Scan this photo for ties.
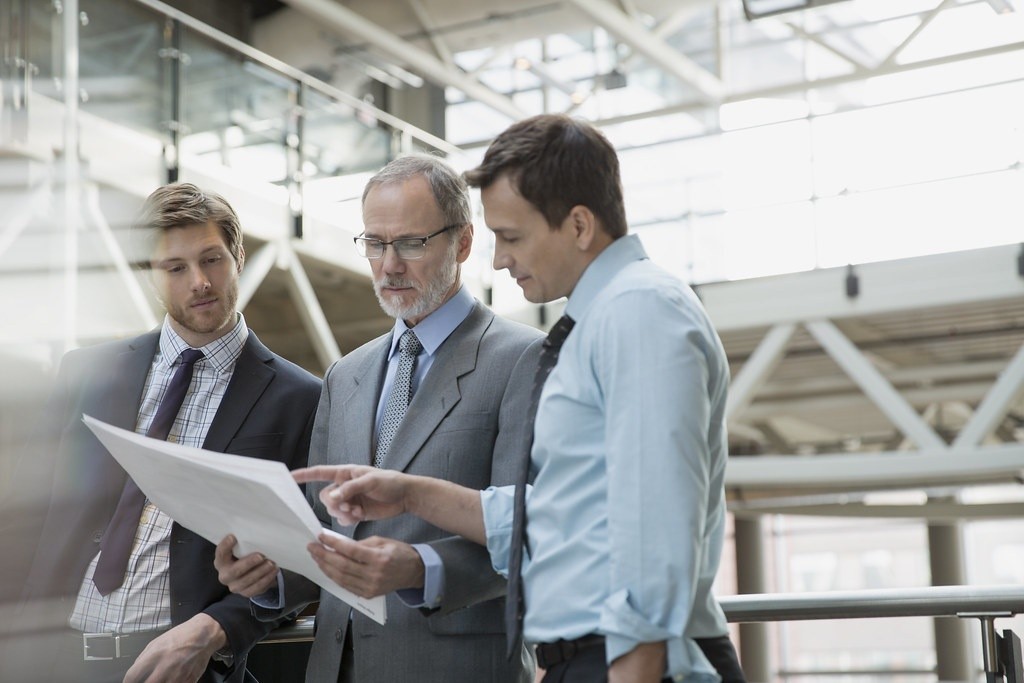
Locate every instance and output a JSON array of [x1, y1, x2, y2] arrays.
[[504, 316, 576, 662], [92, 349, 206, 598], [371, 329, 423, 468]]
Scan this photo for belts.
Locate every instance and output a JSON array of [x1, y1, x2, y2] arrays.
[[59, 628, 172, 663], [536, 637, 605, 668]]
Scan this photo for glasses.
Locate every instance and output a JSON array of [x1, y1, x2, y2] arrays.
[[355, 223, 458, 259]]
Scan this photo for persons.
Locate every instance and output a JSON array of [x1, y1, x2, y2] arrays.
[[214, 154, 550, 682], [290, 113, 741, 682], [23, 113, 323, 683]]
